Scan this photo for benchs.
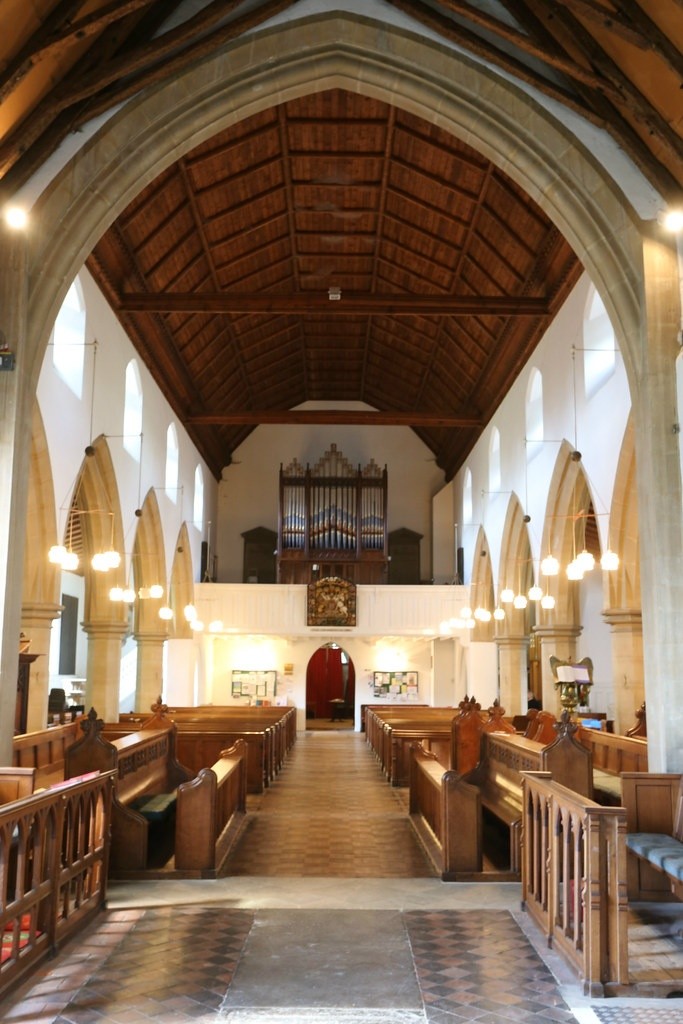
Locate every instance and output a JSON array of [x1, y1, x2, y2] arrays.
[[363, 706, 514, 786], [0, 695, 195, 883], [450, 694, 647, 872], [619, 772, 683, 902], [77, 706, 297, 793]]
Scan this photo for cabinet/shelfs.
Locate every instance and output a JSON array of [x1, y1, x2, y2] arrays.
[[240, 526, 277, 584], [389, 527, 425, 585]]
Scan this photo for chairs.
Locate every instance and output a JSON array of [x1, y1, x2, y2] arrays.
[[48, 688, 68, 725]]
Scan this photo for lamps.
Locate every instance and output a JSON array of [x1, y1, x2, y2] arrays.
[[44, 337, 205, 635], [441, 343, 622, 634]]
